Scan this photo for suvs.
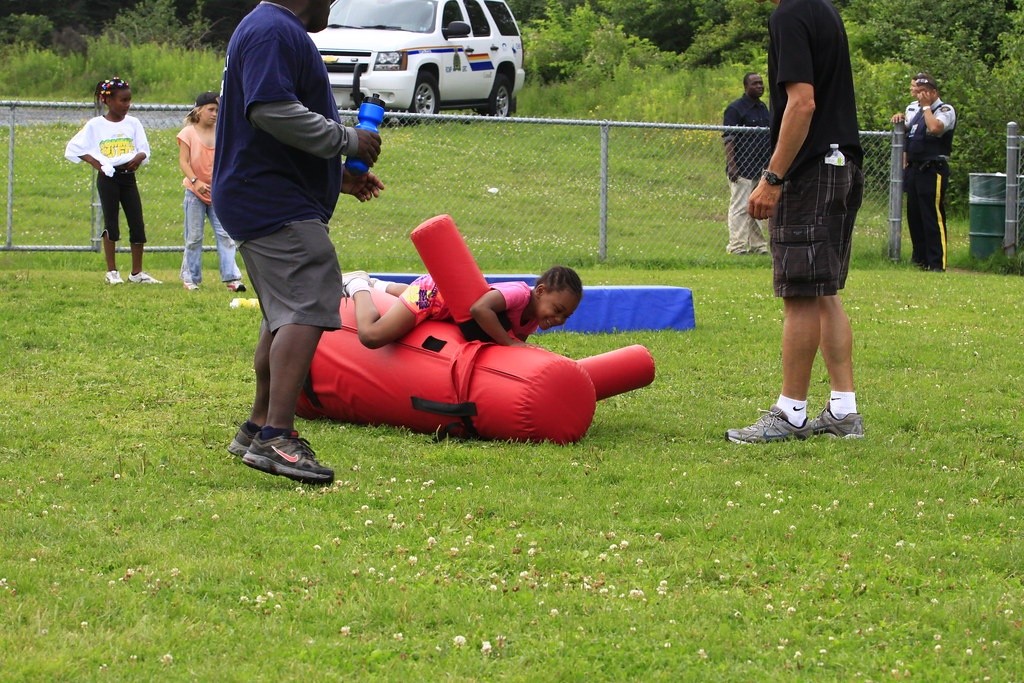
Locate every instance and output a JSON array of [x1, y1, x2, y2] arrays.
[[306, 1, 525, 125]]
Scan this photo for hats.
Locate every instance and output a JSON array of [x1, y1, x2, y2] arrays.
[[186, 91, 221, 117]]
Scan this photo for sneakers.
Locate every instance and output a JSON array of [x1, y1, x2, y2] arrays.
[[105, 270, 125, 286], [342, 271, 370, 296], [226, 280, 246, 292], [183, 281, 200, 290], [227, 422, 256, 457], [810, 400, 865, 439], [724, 403, 814, 443], [242, 428, 335, 486], [126, 272, 163, 285]]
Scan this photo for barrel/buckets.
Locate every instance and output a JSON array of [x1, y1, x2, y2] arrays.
[[969, 173, 1024, 262]]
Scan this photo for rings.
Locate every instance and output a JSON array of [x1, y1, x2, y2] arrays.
[[201, 189, 206, 195]]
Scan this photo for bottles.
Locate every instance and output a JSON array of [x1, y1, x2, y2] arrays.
[[825, 145, 845, 167], [230, 298, 260, 309], [344, 93, 385, 174]]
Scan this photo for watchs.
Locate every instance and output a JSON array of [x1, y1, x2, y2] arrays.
[[191, 177, 198, 183], [762, 170, 785, 186]]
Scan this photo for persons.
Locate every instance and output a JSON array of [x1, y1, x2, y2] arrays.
[[723, 71, 773, 255], [342, 266, 584, 350], [177, 90, 247, 292], [210, 0, 385, 483], [65, 76, 162, 284], [722, 0, 870, 446], [889, 73, 955, 271]]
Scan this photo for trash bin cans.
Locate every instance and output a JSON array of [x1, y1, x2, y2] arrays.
[[968, 168, 1021, 272]]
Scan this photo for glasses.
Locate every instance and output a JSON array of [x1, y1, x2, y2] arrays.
[[916, 79, 936, 90]]
